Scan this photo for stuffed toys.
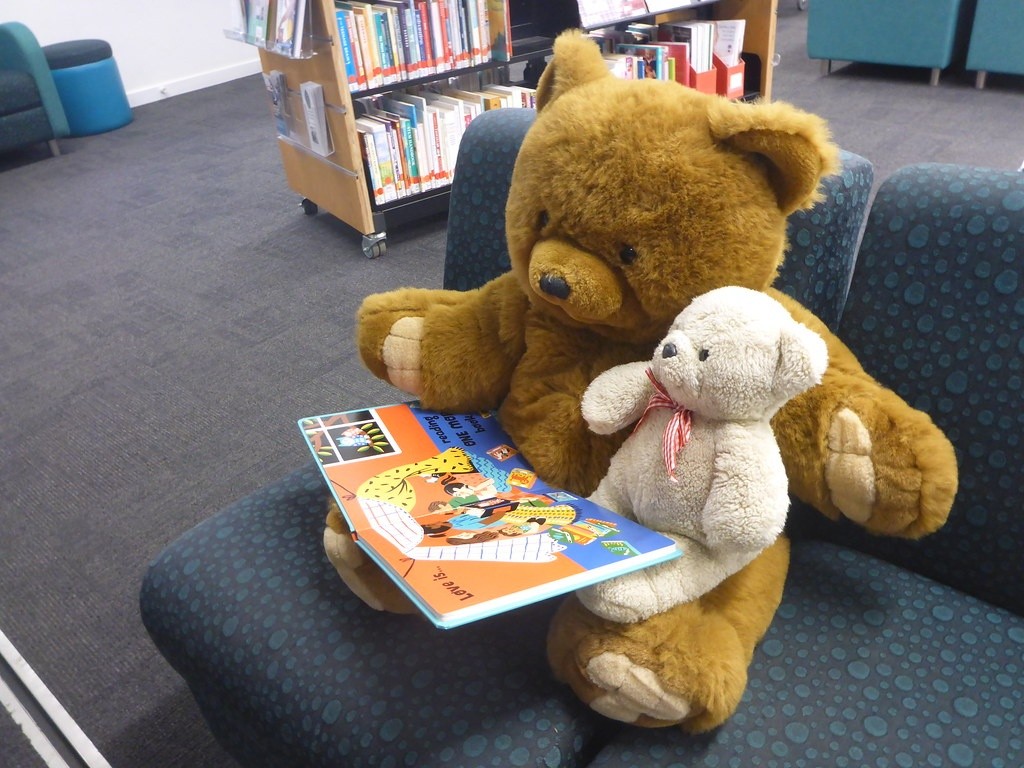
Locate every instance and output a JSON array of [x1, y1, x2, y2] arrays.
[[570, 287, 827, 623], [323, 26, 960, 736]]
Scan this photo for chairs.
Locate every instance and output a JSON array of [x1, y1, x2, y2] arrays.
[[1, 22, 72, 158], [806, 0, 1024, 92], [139, 106, 1024, 764]]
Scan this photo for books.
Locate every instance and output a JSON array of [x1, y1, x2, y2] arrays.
[[335, 0, 745, 207], [297, 399, 682, 631]]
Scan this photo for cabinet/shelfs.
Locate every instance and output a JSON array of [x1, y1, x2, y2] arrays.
[[239, 1, 786, 259]]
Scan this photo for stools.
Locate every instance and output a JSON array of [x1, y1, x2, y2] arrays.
[[40, 39, 134, 139]]
